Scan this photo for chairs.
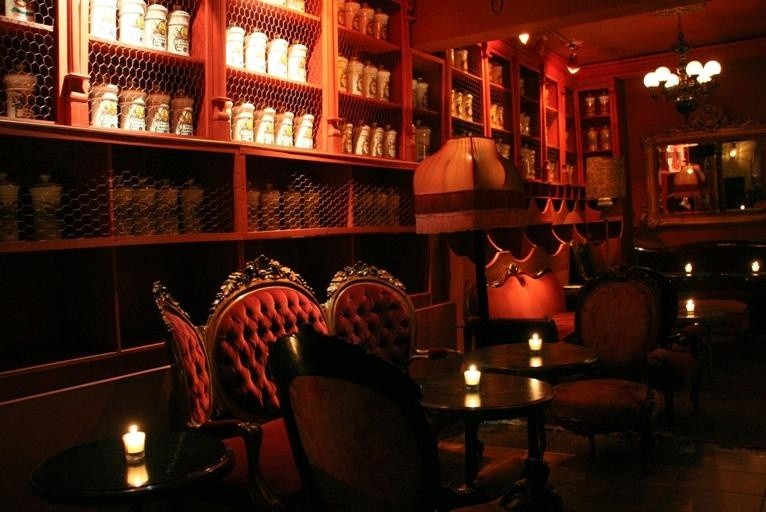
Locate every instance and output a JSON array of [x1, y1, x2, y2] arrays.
[[150, 278, 304, 511], [544, 264, 678, 481], [203, 253, 329, 422], [265, 324, 549, 512], [323, 260, 464, 381]]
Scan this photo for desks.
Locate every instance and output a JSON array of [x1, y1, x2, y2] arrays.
[[462, 338, 605, 378], [675, 297, 749, 386], [28, 430, 235, 511], [412, 369, 556, 491]]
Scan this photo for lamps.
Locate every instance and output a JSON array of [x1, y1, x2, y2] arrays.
[[642, 0, 722, 114], [412, 135, 544, 345]]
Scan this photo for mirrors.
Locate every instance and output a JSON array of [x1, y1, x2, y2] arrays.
[[640, 101, 766, 235]]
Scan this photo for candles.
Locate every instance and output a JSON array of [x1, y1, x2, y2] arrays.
[[528, 339, 543, 351], [750, 259, 759, 272], [121, 429, 146, 454], [463, 370, 482, 385], [683, 259, 692, 272], [683, 296, 696, 313]]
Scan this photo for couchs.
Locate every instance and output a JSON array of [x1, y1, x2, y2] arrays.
[[634, 241, 765, 298]]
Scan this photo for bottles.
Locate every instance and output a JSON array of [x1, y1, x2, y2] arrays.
[[91, 83, 197, 136], [226, 21, 310, 82], [3, 63, 39, 120], [451, 46, 612, 184], [88, 0, 191, 57], [336, 51, 395, 100], [3, 1, 37, 23], [230, 99, 317, 150], [340, 113, 399, 160], [337, 2, 392, 39], [413, 77, 432, 163]]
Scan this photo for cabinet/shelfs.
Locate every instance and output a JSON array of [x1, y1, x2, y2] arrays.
[[0, 0, 629, 378]]
